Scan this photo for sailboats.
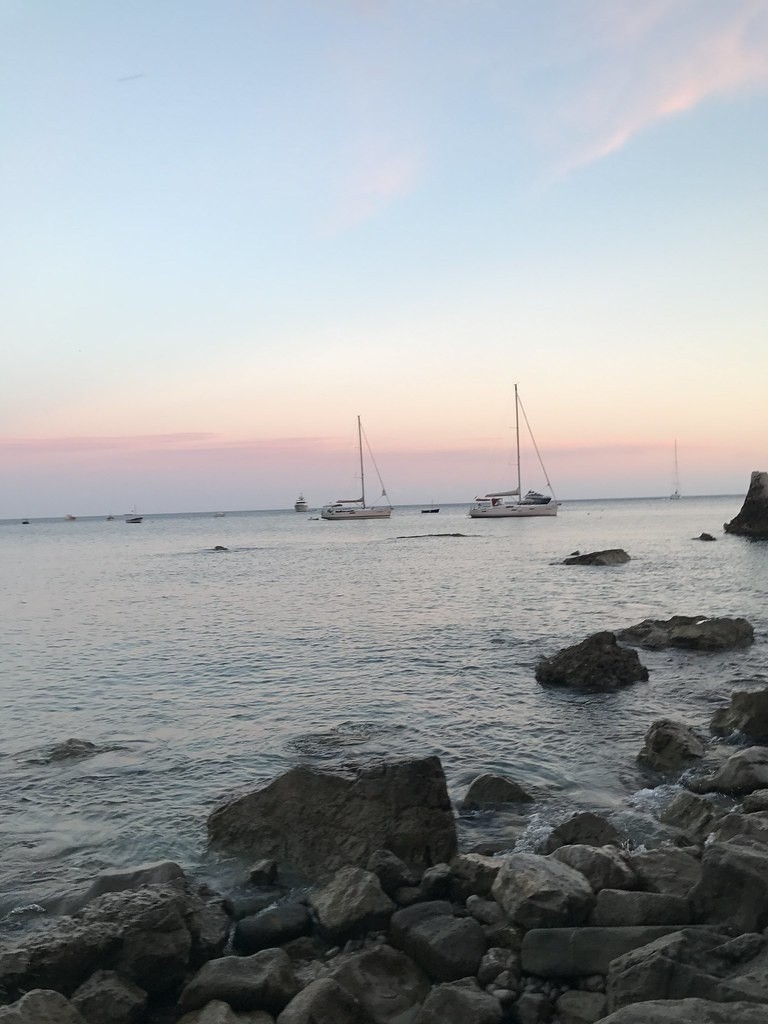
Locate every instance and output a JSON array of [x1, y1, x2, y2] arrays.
[[321, 417, 395, 521], [468, 382, 561, 518]]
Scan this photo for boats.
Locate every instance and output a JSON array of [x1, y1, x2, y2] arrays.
[[421, 507, 440, 513], [126, 517, 143, 524]]
